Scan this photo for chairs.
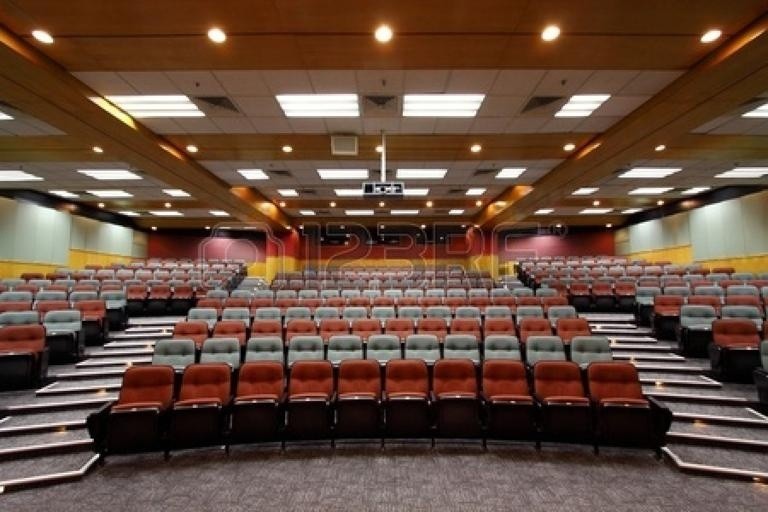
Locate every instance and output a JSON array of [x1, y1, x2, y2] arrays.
[[0, 255, 768, 466]]
